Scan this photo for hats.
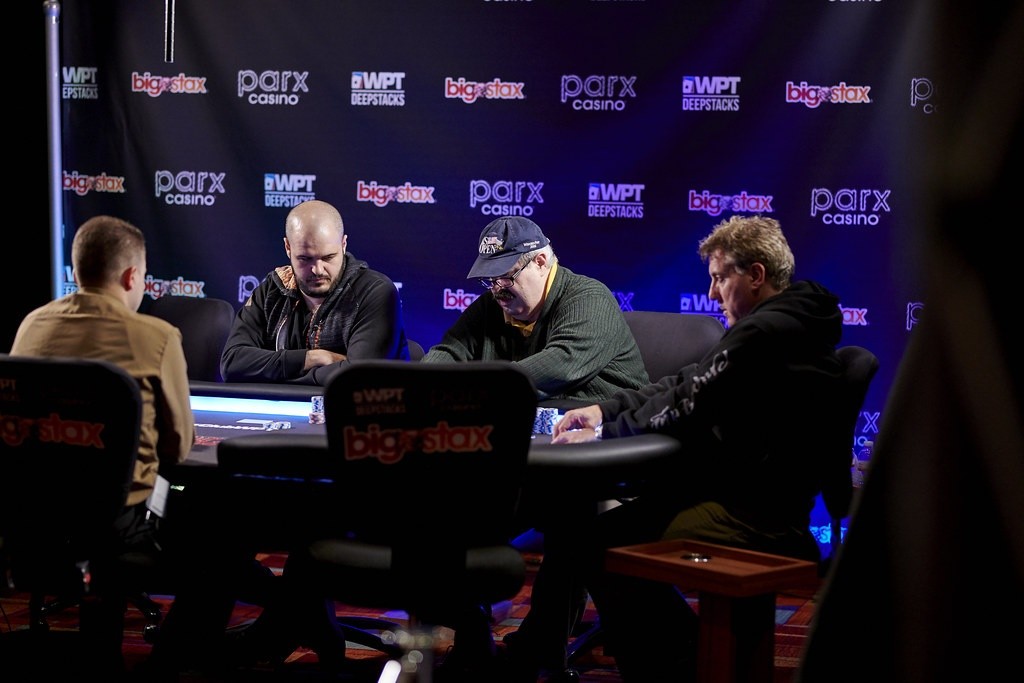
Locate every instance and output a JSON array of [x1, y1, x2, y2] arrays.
[[467, 216, 550, 279]]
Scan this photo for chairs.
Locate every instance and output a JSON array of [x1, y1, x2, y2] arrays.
[[0, 295, 881, 683]]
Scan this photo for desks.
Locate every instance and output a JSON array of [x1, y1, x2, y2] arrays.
[[164, 381, 684, 683], [606, 535, 820, 683]]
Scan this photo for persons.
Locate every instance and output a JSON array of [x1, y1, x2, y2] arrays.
[[552, 215, 843, 683], [419, 216, 651, 683], [7, 215, 247, 683], [797, 0, 1023, 683], [219, 199, 412, 673]]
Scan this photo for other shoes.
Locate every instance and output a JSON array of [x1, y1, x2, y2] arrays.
[[236, 598, 303, 662], [77, 611, 129, 682], [432, 622, 498, 683], [477, 612, 568, 683], [143, 584, 226, 642]]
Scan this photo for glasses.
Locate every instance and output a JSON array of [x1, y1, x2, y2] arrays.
[[479, 258, 532, 290]]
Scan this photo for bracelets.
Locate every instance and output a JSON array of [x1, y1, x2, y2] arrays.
[[595, 425, 602, 439]]
